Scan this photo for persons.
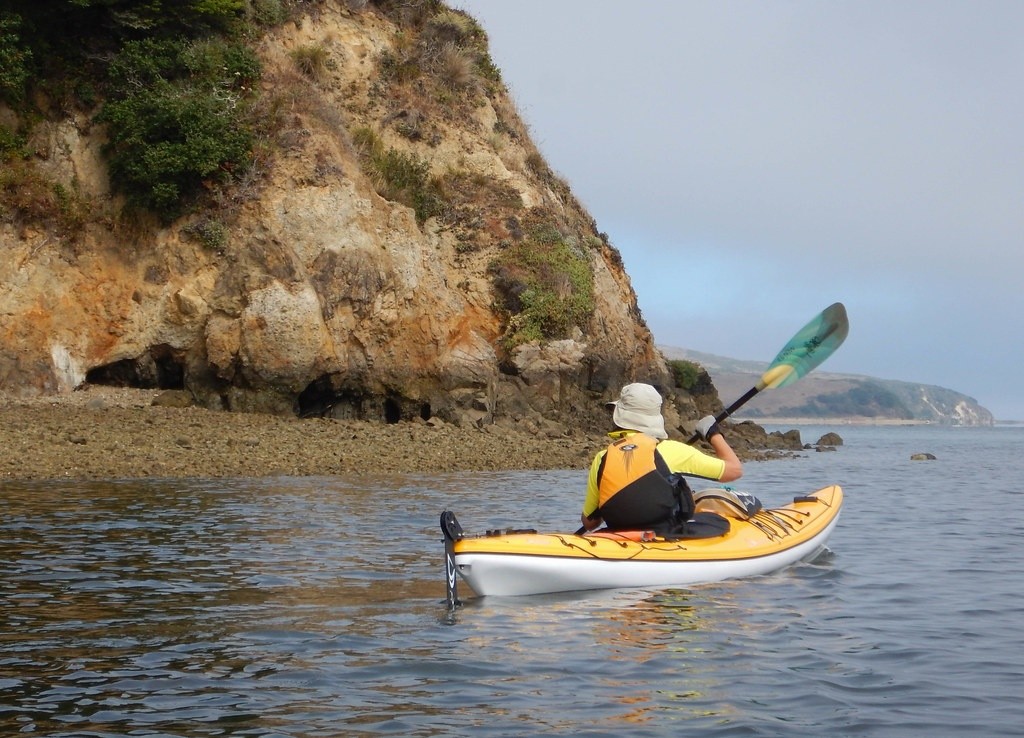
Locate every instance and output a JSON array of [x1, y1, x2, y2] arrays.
[[581, 382, 743, 535]]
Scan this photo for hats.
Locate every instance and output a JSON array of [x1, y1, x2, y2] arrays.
[[605, 383, 669, 439]]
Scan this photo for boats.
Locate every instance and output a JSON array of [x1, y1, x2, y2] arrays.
[[434, 482, 846, 610]]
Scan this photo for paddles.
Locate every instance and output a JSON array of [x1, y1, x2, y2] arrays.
[[577, 303, 851, 536]]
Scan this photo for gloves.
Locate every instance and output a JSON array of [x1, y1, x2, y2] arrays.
[[695, 415, 724, 445]]
